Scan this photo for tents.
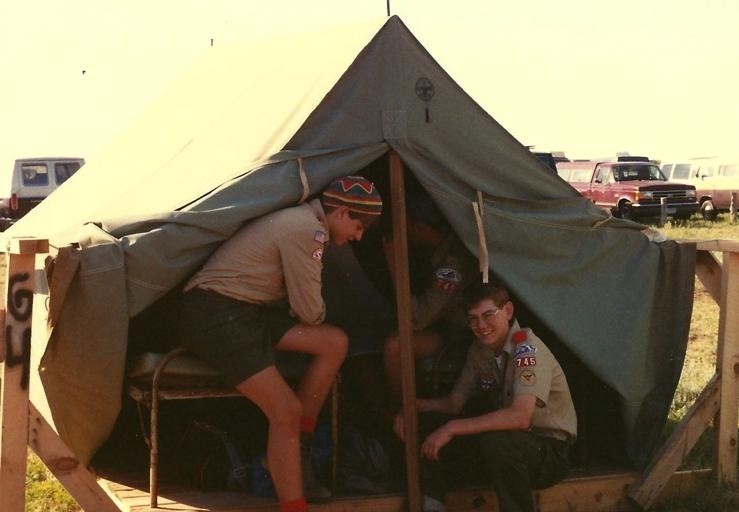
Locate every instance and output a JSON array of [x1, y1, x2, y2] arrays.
[[0, 14, 693, 494]]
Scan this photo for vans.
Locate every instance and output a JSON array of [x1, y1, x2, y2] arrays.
[[9, 156, 85, 216]]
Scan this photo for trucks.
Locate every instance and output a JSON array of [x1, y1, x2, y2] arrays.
[[527, 147, 739, 226]]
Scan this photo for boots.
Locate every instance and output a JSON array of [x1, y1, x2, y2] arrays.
[[299, 444, 333, 503]]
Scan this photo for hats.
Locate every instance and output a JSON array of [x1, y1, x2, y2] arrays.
[[320, 175, 386, 217]]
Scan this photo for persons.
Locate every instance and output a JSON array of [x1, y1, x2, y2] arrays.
[[382, 196, 480, 399], [394, 281, 578, 511], [179, 173, 382, 510]]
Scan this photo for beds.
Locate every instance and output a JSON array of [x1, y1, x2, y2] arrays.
[[120, 337, 343, 509]]
